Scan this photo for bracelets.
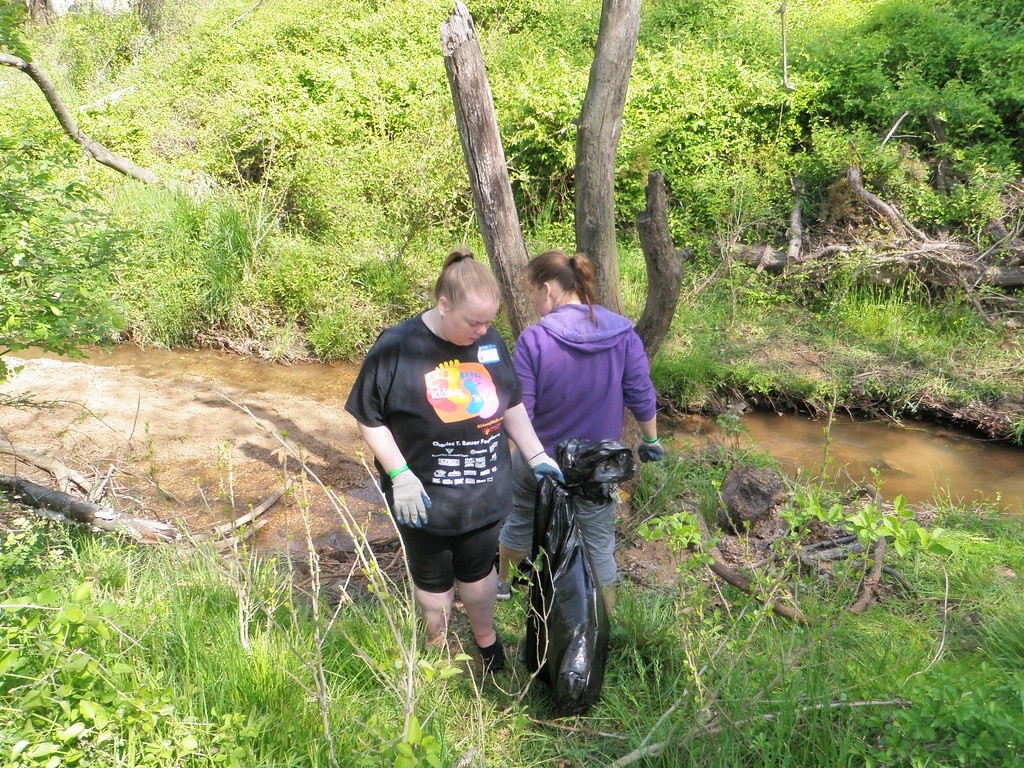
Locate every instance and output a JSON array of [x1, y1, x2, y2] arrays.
[[641, 436, 658, 443], [390, 466, 408, 479]]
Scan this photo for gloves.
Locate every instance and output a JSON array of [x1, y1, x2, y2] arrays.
[[637, 436, 664, 463], [528, 451, 566, 486], [391, 469, 432, 528]]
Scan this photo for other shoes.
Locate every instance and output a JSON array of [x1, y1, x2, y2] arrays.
[[496, 578, 513, 600], [416, 637, 446, 667], [474, 633, 504, 674]]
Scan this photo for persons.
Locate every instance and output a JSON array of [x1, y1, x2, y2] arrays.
[[345, 247, 566, 673], [497, 251, 665, 637]]
